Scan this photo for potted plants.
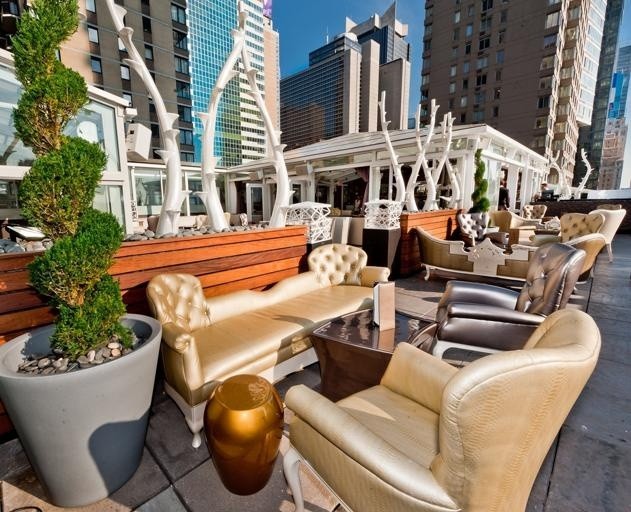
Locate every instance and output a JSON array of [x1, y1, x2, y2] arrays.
[[0, 0, 163, 512]]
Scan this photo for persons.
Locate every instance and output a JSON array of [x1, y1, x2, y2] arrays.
[[540, 182, 548, 195], [499, 180, 510, 211]]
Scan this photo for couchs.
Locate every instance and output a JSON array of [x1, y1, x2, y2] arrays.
[[145, 241, 396, 450]]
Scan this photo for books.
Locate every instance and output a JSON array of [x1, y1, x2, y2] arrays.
[[372, 280, 395, 332]]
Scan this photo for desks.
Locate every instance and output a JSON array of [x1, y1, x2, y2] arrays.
[[309, 305, 440, 404]]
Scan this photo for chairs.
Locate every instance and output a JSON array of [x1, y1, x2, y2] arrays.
[[416, 202, 629, 300], [281, 310, 601, 510], [427, 242, 585, 368]]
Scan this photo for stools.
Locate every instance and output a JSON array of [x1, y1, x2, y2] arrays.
[[201, 375, 286, 497]]
